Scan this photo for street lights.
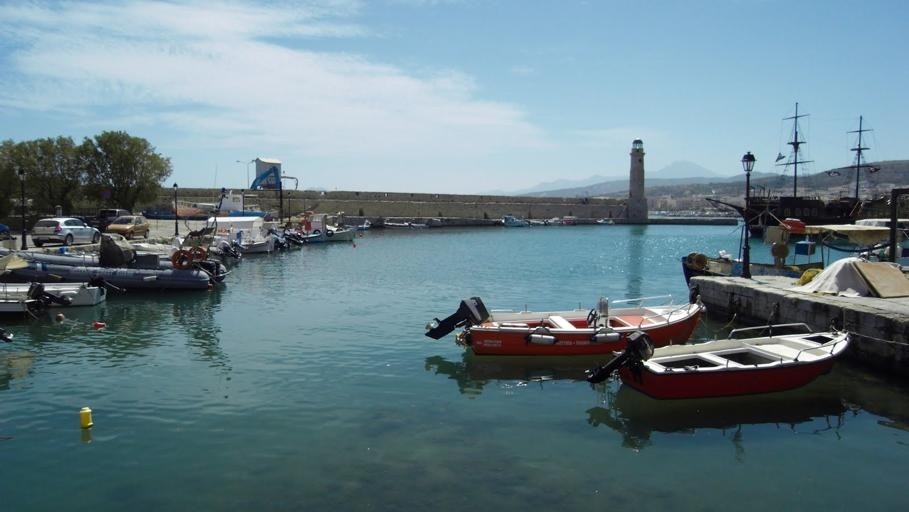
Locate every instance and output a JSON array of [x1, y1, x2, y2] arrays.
[[173, 183, 178, 235], [18, 170, 27, 249], [741, 153, 756, 278]]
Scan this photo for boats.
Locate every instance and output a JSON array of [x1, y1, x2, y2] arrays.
[[683, 241, 824, 290], [382, 220, 427, 227], [243, 209, 370, 252], [423, 289, 706, 357], [596, 217, 616, 224], [0, 233, 241, 313], [585, 320, 851, 399], [502, 214, 562, 226]]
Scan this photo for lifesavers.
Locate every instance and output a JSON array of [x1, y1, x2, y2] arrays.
[[172, 250, 193, 270], [189, 247, 205, 261]]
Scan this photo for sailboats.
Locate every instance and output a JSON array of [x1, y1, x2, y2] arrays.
[[706, 101, 908, 237]]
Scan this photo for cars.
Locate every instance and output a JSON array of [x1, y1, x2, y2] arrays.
[[32, 216, 101, 246], [106, 215, 150, 239]]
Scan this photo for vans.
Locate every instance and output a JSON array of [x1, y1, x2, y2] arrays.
[[99, 208, 131, 223]]
